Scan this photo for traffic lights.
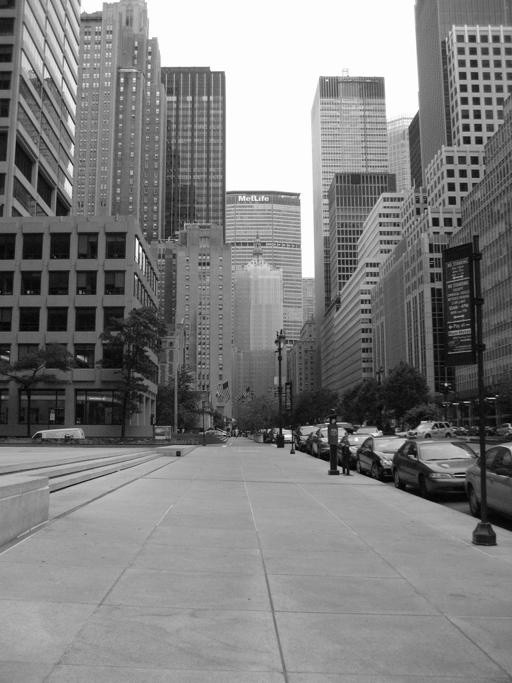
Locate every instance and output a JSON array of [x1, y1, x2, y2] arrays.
[[439, 382, 452, 395]]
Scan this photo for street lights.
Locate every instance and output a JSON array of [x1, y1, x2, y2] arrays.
[[274, 329, 286, 448], [375, 365, 384, 386]]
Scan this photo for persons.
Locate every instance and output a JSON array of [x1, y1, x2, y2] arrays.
[[296, 427, 302, 442], [315, 428, 324, 438], [308, 431, 316, 440], [269, 428, 276, 442], [339, 431, 352, 475]]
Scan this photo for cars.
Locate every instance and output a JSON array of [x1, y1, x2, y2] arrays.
[[198, 428, 227, 437], [263, 419, 512, 521]]
